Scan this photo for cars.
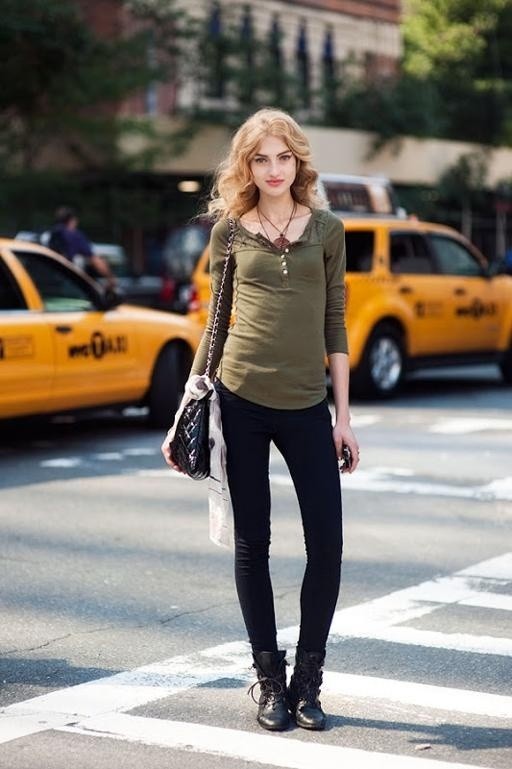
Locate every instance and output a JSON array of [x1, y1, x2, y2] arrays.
[[0, 237, 201, 431]]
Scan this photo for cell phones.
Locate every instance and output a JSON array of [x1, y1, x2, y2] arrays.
[[343, 446, 353, 469]]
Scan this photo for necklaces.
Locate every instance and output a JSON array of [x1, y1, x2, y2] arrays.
[[256, 202, 297, 238], [257, 201, 296, 253]]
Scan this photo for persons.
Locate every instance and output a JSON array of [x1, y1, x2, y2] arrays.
[[37, 206, 120, 291], [161, 215, 209, 319], [161, 110, 360, 733]]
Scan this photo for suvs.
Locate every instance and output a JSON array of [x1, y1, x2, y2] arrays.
[[191, 218, 511, 398]]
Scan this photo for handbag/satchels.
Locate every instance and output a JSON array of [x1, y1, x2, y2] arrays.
[[169, 392, 215, 482]]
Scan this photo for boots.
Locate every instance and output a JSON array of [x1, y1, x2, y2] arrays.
[[252, 650, 289, 731], [289, 647, 325, 730]]
